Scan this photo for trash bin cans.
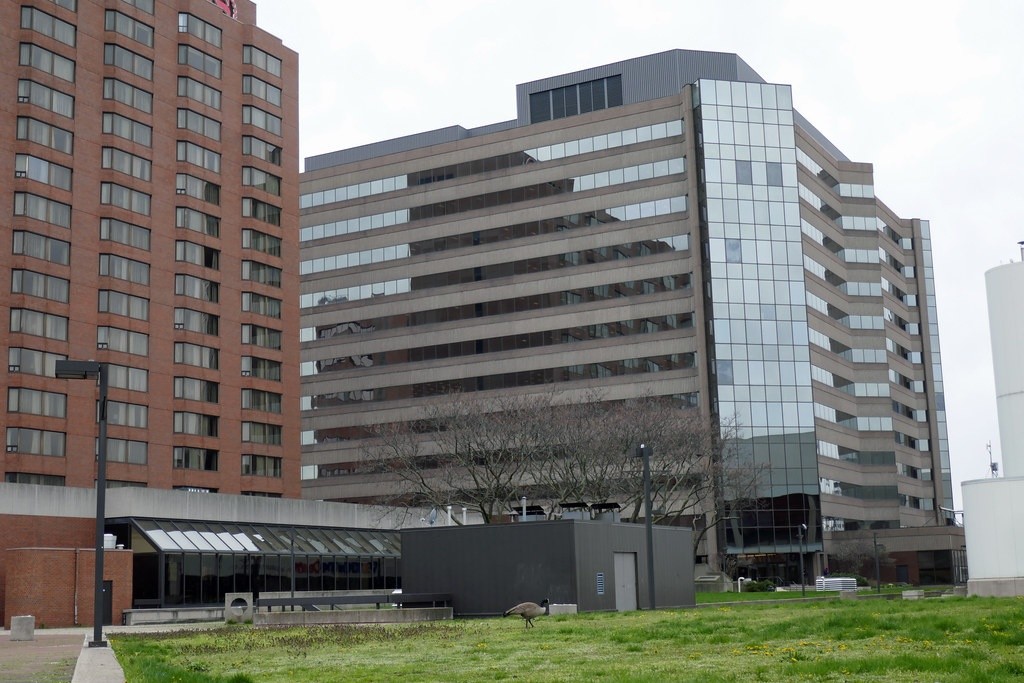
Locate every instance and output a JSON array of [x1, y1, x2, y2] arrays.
[[10, 615, 35, 641], [391, 589, 402, 607]]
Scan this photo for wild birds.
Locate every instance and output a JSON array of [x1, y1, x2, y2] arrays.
[[503, 598, 549, 628]]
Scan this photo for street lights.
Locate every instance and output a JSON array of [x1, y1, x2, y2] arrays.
[[279, 526, 295, 597], [636, 443, 656, 610], [798, 523, 808, 596], [54, 359, 111, 647], [874, 532, 880, 592]]
